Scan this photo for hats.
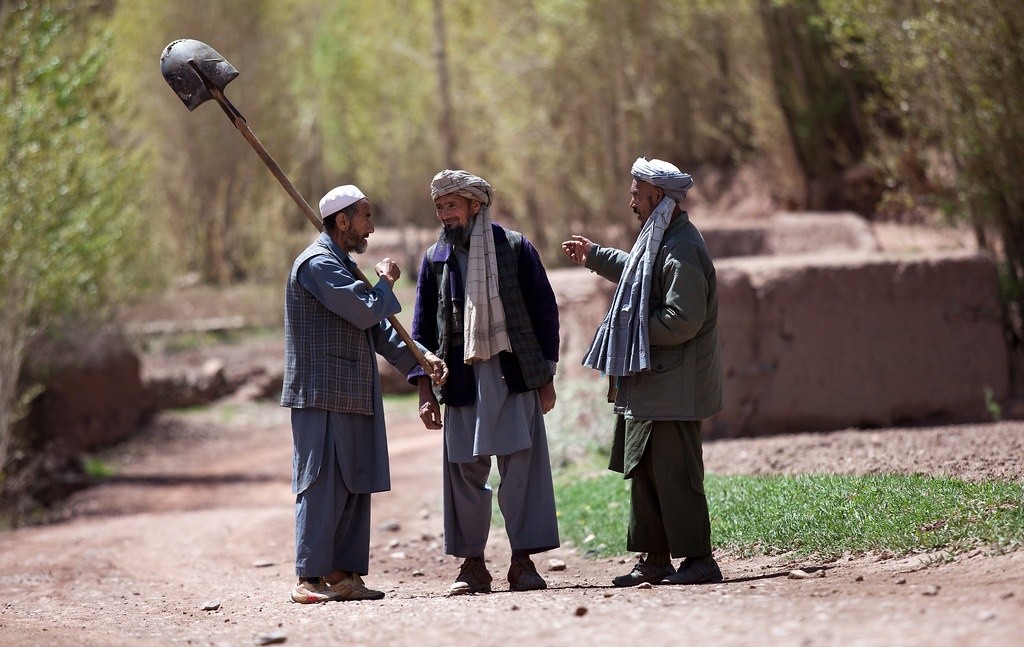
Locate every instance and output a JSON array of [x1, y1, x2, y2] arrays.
[[318, 184, 366, 219]]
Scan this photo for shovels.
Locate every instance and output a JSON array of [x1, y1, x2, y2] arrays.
[[159, 38, 440, 384]]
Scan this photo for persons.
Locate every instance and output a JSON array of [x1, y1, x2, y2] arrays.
[[407, 170, 560, 598], [280, 185, 449, 603], [562, 157, 723, 587]]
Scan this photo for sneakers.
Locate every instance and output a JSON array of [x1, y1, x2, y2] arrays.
[[330, 572, 385, 601], [659, 554, 724, 585], [612, 554, 676, 587], [289, 581, 342, 605], [506, 558, 548, 590], [449, 560, 493, 593]]
